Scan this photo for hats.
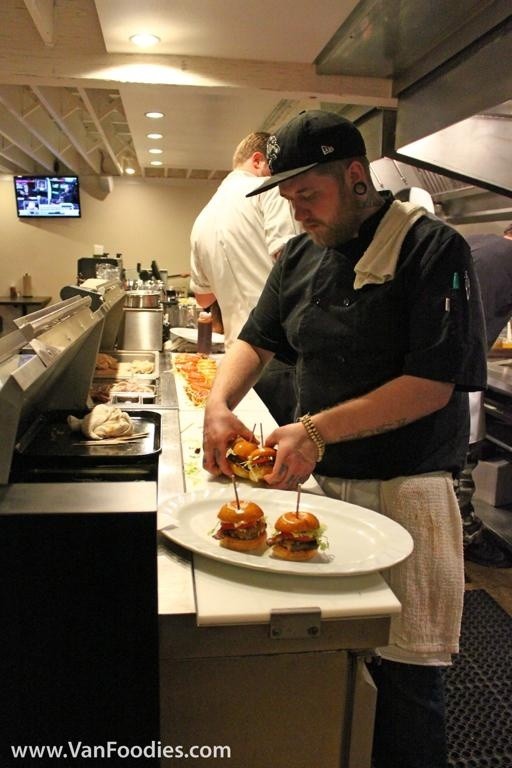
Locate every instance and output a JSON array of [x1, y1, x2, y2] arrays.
[[245, 110, 365, 197]]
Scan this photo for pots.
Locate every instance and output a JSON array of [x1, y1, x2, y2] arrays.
[[123, 290, 196, 328]]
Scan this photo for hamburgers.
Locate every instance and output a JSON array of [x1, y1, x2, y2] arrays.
[[227, 438, 258, 478], [208, 500, 267, 556], [266, 512, 329, 561], [241, 447, 277, 483]]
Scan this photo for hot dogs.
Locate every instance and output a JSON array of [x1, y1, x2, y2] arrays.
[[100, 381, 156, 395]]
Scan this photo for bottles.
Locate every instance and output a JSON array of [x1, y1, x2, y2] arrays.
[[197, 312, 212, 353], [9, 271, 32, 300]]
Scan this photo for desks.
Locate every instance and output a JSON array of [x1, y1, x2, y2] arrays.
[[0, 296, 51, 315]]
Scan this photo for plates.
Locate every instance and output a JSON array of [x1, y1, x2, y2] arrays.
[[157, 488, 414, 577], [170, 327, 226, 345]]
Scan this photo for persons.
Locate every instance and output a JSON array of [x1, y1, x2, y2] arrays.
[[451, 220, 512, 569], [189, 131, 309, 426], [202, 110, 488, 768]]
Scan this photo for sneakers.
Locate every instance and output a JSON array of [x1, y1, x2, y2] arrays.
[[463, 525, 512, 569]]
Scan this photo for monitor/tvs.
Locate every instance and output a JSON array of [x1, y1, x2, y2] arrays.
[[14, 176, 81, 218]]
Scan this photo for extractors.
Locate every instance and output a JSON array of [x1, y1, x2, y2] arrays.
[[358, 116, 489, 199]]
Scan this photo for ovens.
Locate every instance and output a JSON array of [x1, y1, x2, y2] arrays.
[[469, 386, 511, 462]]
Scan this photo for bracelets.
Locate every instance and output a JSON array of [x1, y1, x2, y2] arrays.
[[299, 414, 326, 462]]
